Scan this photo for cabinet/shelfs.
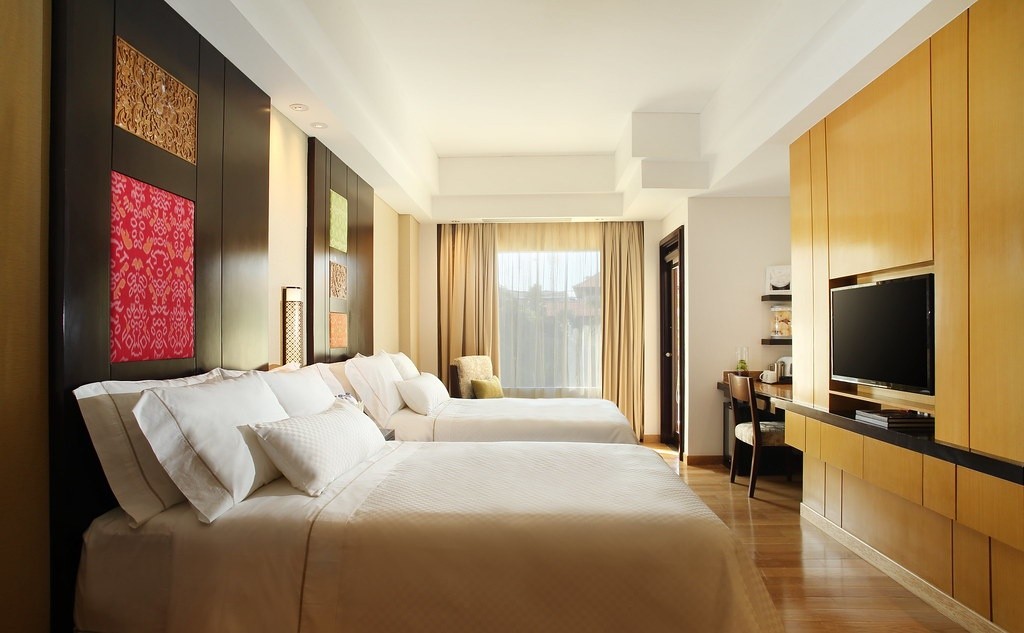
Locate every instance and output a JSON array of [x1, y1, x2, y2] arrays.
[[786, 0, 1024, 633]]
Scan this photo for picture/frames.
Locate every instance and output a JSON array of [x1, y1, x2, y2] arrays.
[[766, 266, 792, 295]]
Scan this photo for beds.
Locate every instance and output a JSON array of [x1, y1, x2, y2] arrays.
[[386, 397, 639, 444], [72, 438, 786, 633]]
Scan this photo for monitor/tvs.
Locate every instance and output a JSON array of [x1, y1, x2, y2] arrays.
[[830, 273, 934, 396]]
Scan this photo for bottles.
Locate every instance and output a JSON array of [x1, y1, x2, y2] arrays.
[[778, 361, 785, 381]]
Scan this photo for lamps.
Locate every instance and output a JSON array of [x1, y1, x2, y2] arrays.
[[285, 286, 305, 367]]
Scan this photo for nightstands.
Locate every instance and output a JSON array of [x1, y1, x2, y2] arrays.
[[380, 428, 395, 441]]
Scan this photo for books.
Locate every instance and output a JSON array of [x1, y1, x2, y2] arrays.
[[855, 409, 935, 431]]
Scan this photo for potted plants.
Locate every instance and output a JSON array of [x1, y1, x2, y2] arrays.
[[737, 360, 749, 377]]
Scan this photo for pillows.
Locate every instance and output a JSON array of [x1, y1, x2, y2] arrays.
[[344, 350, 406, 429], [247, 396, 388, 496], [388, 351, 421, 380], [220, 361, 301, 379], [73, 368, 223, 529], [320, 361, 358, 402], [471, 375, 504, 399], [257, 362, 337, 419], [132, 369, 292, 524], [395, 372, 450, 415]]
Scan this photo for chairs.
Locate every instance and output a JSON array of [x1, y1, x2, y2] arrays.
[[728, 372, 803, 498], [449, 355, 493, 399]]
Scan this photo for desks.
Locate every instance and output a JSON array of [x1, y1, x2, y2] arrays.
[[717, 371, 793, 475]]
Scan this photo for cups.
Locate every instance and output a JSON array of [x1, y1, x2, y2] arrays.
[[760, 370, 770, 382], [766, 371, 775, 383]]
